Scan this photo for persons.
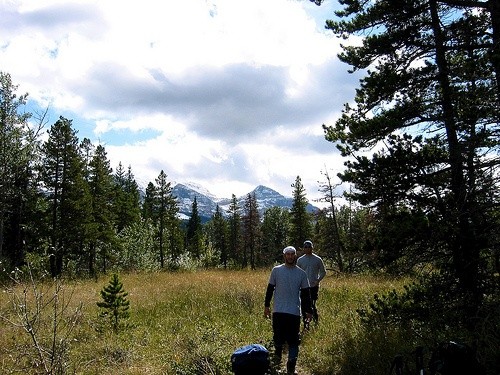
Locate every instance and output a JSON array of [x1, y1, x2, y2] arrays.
[[297, 240, 327, 333], [264, 246, 312, 375]]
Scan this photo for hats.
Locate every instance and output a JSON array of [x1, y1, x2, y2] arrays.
[[230, 344, 271, 372], [283, 246, 296, 254], [303, 240, 312, 246]]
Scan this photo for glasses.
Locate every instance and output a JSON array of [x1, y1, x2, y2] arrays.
[[304, 245, 312, 248]]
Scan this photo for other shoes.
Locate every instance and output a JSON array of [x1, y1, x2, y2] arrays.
[[287, 370, 298, 375], [275, 349, 282, 362]]
